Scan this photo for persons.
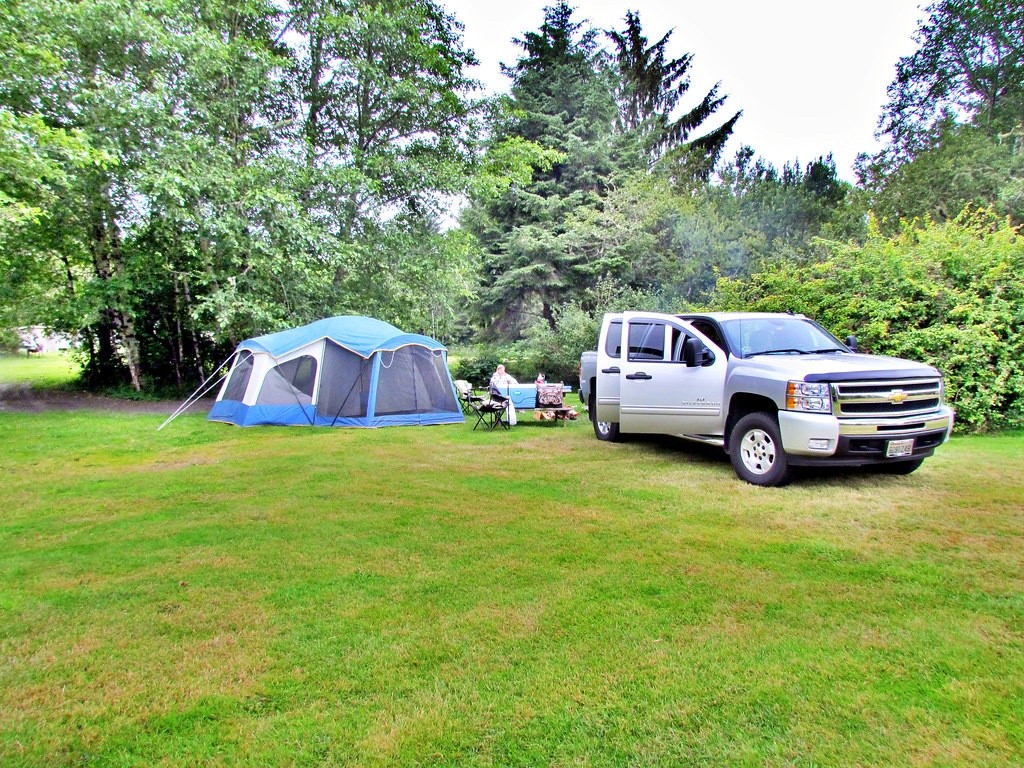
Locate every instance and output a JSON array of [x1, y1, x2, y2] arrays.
[[490, 364, 517, 383]]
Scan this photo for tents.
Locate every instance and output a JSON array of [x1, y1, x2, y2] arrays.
[[209, 315, 466, 428]]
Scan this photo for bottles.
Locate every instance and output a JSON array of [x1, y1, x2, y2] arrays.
[[537, 373, 545, 380]]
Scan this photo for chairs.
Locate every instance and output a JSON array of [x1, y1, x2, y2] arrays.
[[453, 380, 490, 413], [468, 386, 511, 431]]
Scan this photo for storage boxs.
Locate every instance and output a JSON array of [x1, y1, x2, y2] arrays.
[[497, 383, 564, 409]]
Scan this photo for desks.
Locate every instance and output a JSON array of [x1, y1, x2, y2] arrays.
[[535, 407, 572, 428]]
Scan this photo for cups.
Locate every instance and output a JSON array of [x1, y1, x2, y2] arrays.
[[537, 379, 543, 384], [545, 381, 547, 384]]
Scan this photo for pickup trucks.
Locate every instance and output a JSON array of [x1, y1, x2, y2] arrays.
[[579, 309, 955, 488]]
